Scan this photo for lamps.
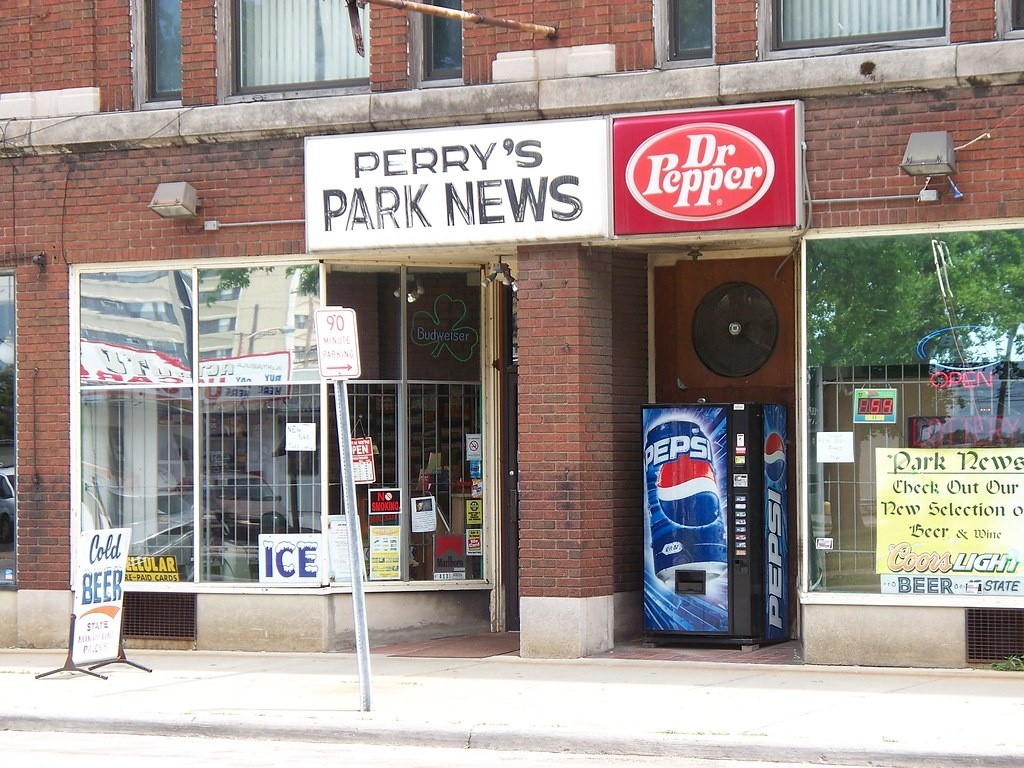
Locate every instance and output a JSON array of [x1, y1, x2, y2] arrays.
[[146, 181, 198, 218], [902, 131, 958, 180], [480, 273, 498, 287]]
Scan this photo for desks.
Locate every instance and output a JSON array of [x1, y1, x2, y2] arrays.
[[425, 494, 473, 579]]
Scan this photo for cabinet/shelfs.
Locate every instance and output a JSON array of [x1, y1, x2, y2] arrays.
[[203, 435, 247, 467]]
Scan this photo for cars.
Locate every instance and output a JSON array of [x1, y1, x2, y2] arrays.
[[211, 471, 290, 531], [0, 439, 15, 543]]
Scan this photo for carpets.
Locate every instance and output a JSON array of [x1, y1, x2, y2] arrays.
[[384, 635, 520, 658]]
